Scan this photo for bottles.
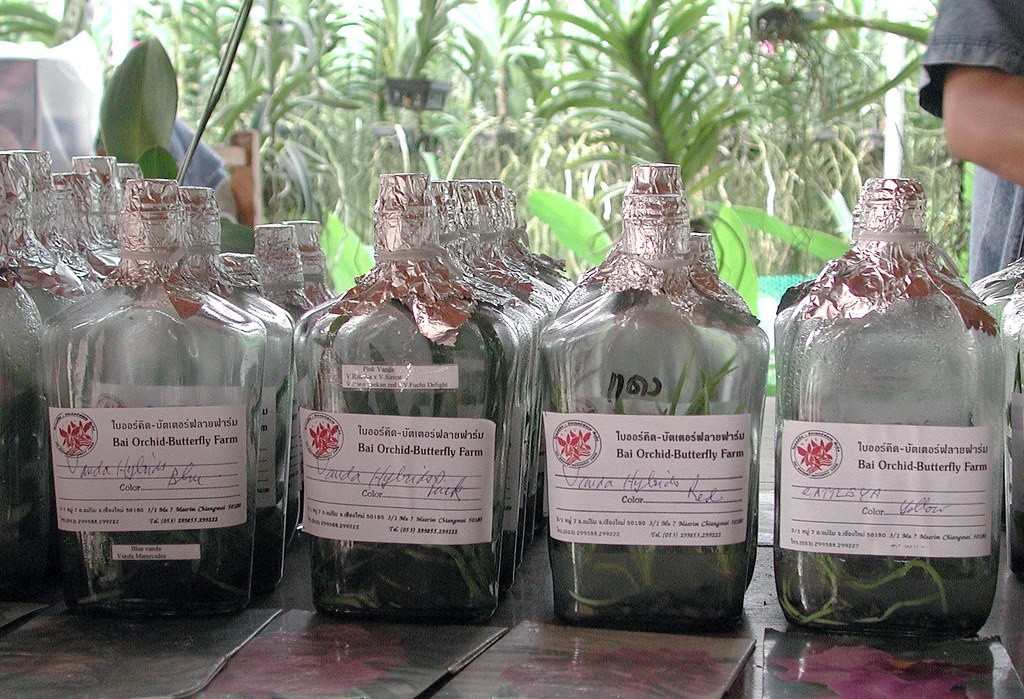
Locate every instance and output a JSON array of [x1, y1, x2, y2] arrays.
[[0, 149, 1024, 636]]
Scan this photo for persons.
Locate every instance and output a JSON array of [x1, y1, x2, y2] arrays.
[[918, 0, 1024, 284]]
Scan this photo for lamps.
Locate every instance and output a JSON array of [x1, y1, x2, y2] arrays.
[[369, 75, 454, 145]]
